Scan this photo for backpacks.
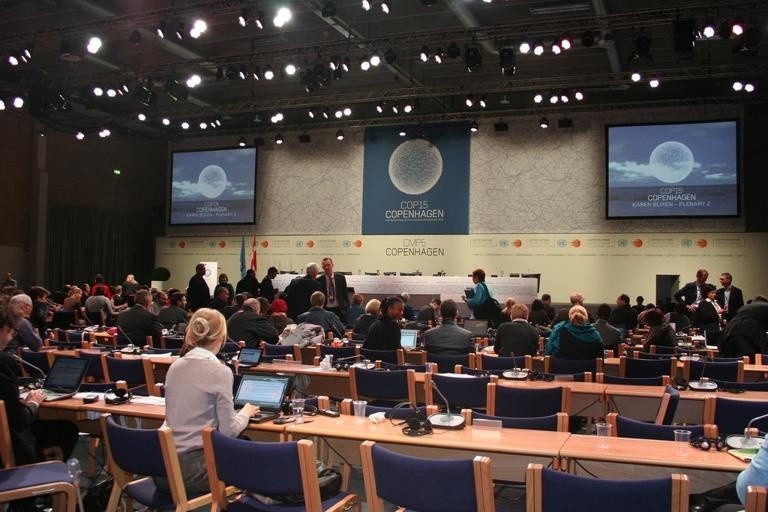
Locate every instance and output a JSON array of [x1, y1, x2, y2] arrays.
[[475, 282, 499, 327]]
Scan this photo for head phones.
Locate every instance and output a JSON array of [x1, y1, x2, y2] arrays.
[[402, 418, 434, 436], [692, 436, 725, 450], [333, 361, 350, 369], [390, 402, 425, 426], [103, 388, 132, 403]]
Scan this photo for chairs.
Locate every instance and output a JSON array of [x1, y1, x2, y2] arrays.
[[221, 341, 245, 353], [355, 346, 403, 365], [755, 354, 768, 365], [476, 353, 533, 372], [705, 356, 750, 364], [348, 365, 417, 408], [633, 351, 686, 361], [422, 351, 475, 370], [522, 369, 592, 382], [544, 356, 604, 374], [745, 485, 768, 512], [101, 354, 163, 397], [619, 357, 677, 380], [596, 372, 671, 386], [640, 386, 681, 426], [0, 399, 82, 512], [650, 345, 679, 354], [89, 342, 135, 350], [66, 331, 94, 342], [605, 412, 718, 442], [684, 359, 744, 383], [341, 398, 439, 420], [486, 383, 571, 418], [461, 408, 569, 433], [425, 372, 499, 418], [75, 349, 106, 383], [261, 341, 303, 364], [161, 336, 184, 349], [17, 347, 55, 378], [44, 339, 90, 351], [316, 344, 356, 366], [703, 397, 768, 438]]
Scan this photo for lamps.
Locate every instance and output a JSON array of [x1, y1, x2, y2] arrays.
[[334, 108, 343, 120], [329, 58, 340, 71], [701, 24, 715, 39], [731, 23, 744, 35], [237, 8, 249, 27], [628, 70, 641, 84], [732, 81, 741, 93], [359, 440, 495, 512], [575, 88, 584, 102], [561, 36, 573, 49], [647, 78, 659, 89], [540, 117, 548, 128], [376, 103, 384, 114], [341, 63, 351, 72], [531, 42, 545, 56], [200, 426, 360, 512], [98, 413, 238, 512], [551, 42, 560, 55], [560, 93, 570, 104], [137, 84, 154, 107], [361, 0, 373, 11], [307, 107, 316, 122], [548, 90, 559, 105], [321, 110, 330, 121], [526, 463, 689, 512], [519, 42, 530, 54], [155, 19, 168, 39], [304, 74, 317, 93], [343, 104, 352, 117], [317, 68, 330, 88], [176, 21, 185, 41], [469, 121, 478, 132], [255, 11, 265, 29], [334, 129, 345, 141], [419, 48, 429, 62], [238, 139, 246, 146], [744, 82, 757, 98], [275, 136, 284, 144], [434, 51, 446, 65], [533, 91, 544, 104]]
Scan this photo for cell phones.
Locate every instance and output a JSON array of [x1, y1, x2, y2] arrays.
[[83, 394, 99, 403], [273, 417, 294, 423]]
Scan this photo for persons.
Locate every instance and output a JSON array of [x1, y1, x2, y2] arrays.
[[529, 300, 547, 327], [260, 267, 278, 302], [149, 288, 180, 313], [592, 304, 622, 355], [123, 274, 140, 300], [54, 284, 71, 304], [399, 293, 416, 322], [114, 286, 124, 306], [495, 304, 539, 358], [610, 295, 637, 326], [92, 274, 111, 300], [699, 286, 727, 346], [84, 287, 113, 324], [542, 294, 557, 324], [297, 291, 345, 337], [156, 293, 188, 327], [715, 273, 743, 325], [190, 263, 209, 313], [0, 301, 81, 466], [268, 300, 294, 335], [675, 303, 691, 332], [64, 285, 82, 308], [26, 285, 49, 327], [242, 292, 252, 299], [417, 298, 441, 326], [224, 295, 247, 318], [215, 273, 234, 301], [259, 297, 269, 318], [116, 290, 160, 348], [638, 308, 677, 358], [558, 292, 595, 323], [463, 269, 493, 319], [675, 271, 715, 325], [289, 264, 320, 313], [425, 299, 476, 354], [208, 287, 230, 317], [347, 294, 364, 329], [353, 299, 380, 341], [4, 295, 42, 354], [227, 298, 278, 348], [363, 296, 403, 351], [543, 306, 601, 371], [153, 310, 260, 498], [236, 269, 259, 305], [721, 296, 768, 364], [689, 432, 767, 512], [81, 284, 90, 306], [501, 299, 515, 318], [631, 296, 645, 313], [0, 272, 17, 293], [318, 258, 347, 323]]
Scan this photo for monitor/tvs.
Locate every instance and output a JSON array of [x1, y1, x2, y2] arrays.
[[463, 319, 488, 336], [400, 328, 417, 350], [605, 119, 741, 220], [171, 150, 254, 224]]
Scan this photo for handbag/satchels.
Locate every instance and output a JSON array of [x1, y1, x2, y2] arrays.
[[474, 283, 499, 319]]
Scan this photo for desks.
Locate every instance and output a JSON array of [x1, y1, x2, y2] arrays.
[[18, 392, 84, 411], [604, 383, 766, 426], [606, 357, 768, 382], [283, 410, 570, 496], [80, 395, 325, 467], [46, 347, 180, 365], [559, 431, 768, 483], [235, 352, 428, 392], [424, 368, 606, 418]]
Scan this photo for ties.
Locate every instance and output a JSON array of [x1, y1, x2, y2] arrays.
[[328, 275, 334, 302]]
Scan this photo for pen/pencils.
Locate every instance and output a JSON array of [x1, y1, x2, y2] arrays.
[[294, 419, 314, 425]]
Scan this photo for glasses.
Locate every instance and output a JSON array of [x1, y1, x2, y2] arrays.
[[10, 326, 19, 336]]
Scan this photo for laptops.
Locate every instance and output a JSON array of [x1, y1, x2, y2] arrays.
[[226, 348, 262, 367], [21, 357, 90, 402], [233, 373, 293, 421]]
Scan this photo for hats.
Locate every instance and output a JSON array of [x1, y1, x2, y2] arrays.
[[271, 299, 288, 313]]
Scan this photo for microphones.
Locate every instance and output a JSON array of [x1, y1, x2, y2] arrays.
[[510, 351, 517, 370], [430, 380, 449, 414], [118, 326, 133, 345]]
